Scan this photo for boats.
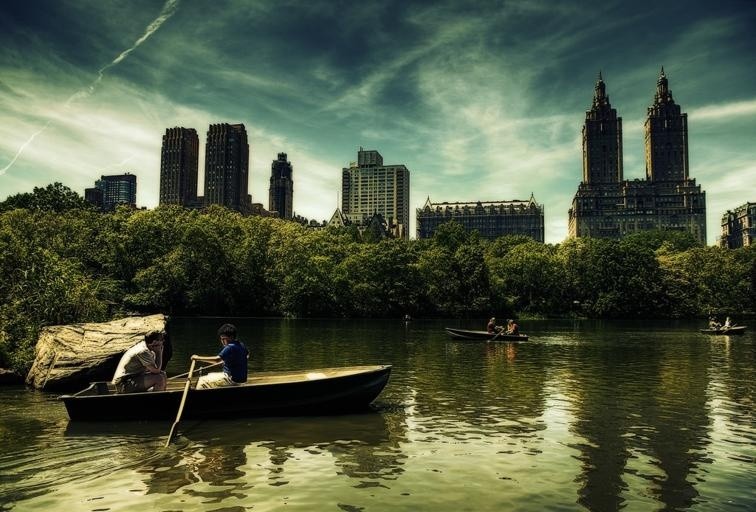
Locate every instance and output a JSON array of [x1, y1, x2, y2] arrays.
[[700, 326, 748, 335], [59, 363, 393, 420], [445, 326, 530, 342]]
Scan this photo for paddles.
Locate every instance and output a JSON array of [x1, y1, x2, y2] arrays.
[[165, 359, 196, 447]]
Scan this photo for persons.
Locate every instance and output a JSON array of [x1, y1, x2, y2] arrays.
[[487, 317, 519, 336], [190, 323, 250, 390], [709, 317, 734, 330], [111, 330, 167, 393]]
